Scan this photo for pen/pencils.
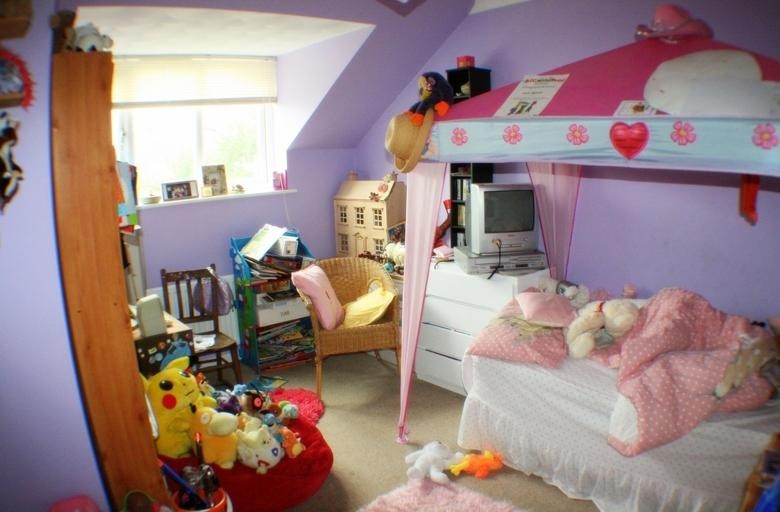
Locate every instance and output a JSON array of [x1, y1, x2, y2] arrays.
[[158, 432, 216, 508]]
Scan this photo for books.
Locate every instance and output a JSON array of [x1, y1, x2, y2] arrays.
[[233, 222, 316, 368]]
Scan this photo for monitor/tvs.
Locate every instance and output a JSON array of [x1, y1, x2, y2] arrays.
[[465, 181, 540, 256]]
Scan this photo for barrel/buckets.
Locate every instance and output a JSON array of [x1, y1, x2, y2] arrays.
[[172, 487, 233, 512]]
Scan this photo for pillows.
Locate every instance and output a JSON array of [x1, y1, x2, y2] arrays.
[[465, 284, 577, 371], [290, 264, 396, 331]]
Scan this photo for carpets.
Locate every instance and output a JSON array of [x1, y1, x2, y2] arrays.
[[355, 473, 531, 512]]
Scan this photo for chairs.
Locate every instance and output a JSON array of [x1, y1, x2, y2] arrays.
[[293, 255, 409, 400], [159, 263, 246, 385]]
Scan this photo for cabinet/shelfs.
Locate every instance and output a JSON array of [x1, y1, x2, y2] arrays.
[[446, 64, 494, 251], [228, 233, 317, 382]]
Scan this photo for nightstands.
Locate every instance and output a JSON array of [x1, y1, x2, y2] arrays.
[[414, 262, 556, 397]]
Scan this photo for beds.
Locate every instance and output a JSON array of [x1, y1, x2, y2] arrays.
[[467, 287, 779, 512]]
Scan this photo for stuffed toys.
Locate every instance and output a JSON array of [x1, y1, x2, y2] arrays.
[[51, 8, 77, 52], [141, 356, 306, 475], [72, 22, 116, 53], [450, 450, 506, 479], [540, 276, 590, 308], [403, 72, 456, 127], [564, 297, 641, 358], [406, 440, 464, 483], [715, 333, 780, 398]]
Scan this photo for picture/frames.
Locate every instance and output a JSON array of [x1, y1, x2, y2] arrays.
[[161, 180, 200, 202], [201, 166, 230, 197]]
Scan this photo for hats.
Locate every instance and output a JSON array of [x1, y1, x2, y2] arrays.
[[634, 3, 714, 40], [383, 107, 434, 173]]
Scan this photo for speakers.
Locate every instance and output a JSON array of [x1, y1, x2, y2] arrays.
[[136, 293, 167, 338]]
